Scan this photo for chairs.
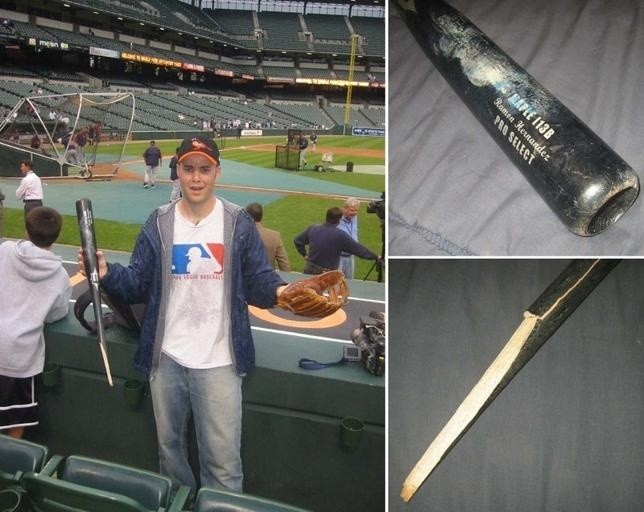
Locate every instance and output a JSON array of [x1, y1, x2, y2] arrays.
[[22, 454, 192, 512], [189, 476, 314, 512], [1, 432, 48, 512]]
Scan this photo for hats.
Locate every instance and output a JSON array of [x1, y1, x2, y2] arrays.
[[178, 136, 220, 166]]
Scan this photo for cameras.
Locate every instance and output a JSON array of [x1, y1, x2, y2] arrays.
[[343, 310, 385, 376], [367, 192, 385, 223]]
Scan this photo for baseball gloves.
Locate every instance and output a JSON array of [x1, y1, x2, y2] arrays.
[[277, 271, 349, 319]]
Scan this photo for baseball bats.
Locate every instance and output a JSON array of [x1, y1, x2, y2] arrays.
[[76, 199, 114, 388], [394, 0, 640, 236]]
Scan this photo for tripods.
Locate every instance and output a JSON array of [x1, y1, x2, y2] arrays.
[[363, 259, 385, 282]]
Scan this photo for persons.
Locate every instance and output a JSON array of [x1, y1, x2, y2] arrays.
[[75, 134, 349, 512], [337, 197, 361, 277], [142, 139, 164, 190], [0, 85, 102, 165], [297, 131, 309, 166], [16, 161, 44, 221], [179, 113, 330, 130], [234, 124, 244, 141], [1, 206, 71, 440], [294, 206, 381, 277], [168, 146, 183, 202], [247, 202, 291, 273], [310, 132, 319, 151]]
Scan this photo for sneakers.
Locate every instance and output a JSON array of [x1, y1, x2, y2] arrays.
[[151, 184, 156, 189], [144, 182, 149, 188]]
